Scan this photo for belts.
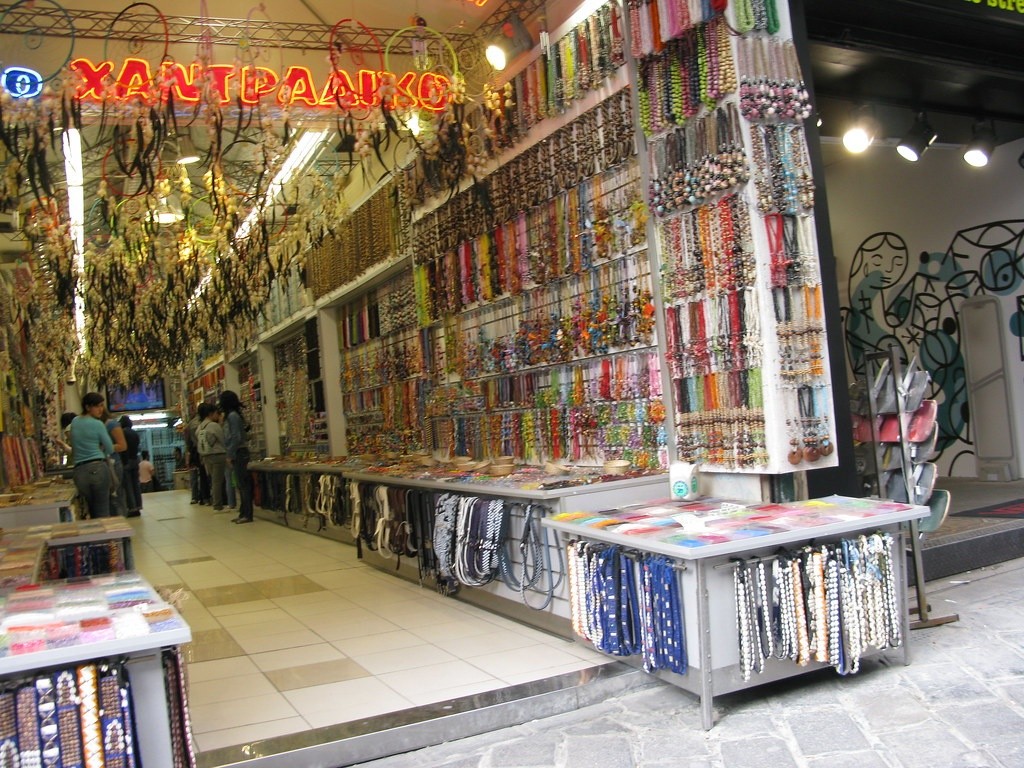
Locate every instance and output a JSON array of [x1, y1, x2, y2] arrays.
[[202, 453, 226, 457], [73, 458, 107, 469]]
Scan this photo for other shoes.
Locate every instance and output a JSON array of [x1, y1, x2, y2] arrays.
[[127, 510, 140, 518], [231, 515, 253, 524]]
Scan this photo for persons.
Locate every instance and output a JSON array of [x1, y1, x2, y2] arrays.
[[55, 392, 144, 521], [186, 390, 254, 525]]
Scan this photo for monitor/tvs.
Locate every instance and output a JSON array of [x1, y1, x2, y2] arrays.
[[106, 378, 166, 412]]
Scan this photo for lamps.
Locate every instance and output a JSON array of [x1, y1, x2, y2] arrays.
[[175, 133, 200, 164], [144, 195, 184, 225], [484, 17, 534, 71]]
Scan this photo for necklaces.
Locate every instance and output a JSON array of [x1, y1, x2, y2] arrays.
[[207, 1, 917, 682], [0, 264, 85, 505]]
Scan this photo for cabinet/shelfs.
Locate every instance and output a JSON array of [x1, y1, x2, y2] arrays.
[[860, 343, 960, 632]]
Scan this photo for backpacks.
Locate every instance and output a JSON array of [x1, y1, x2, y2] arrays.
[[198, 421, 216, 454]]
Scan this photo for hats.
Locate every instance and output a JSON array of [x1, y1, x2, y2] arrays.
[[117, 416, 133, 427]]
